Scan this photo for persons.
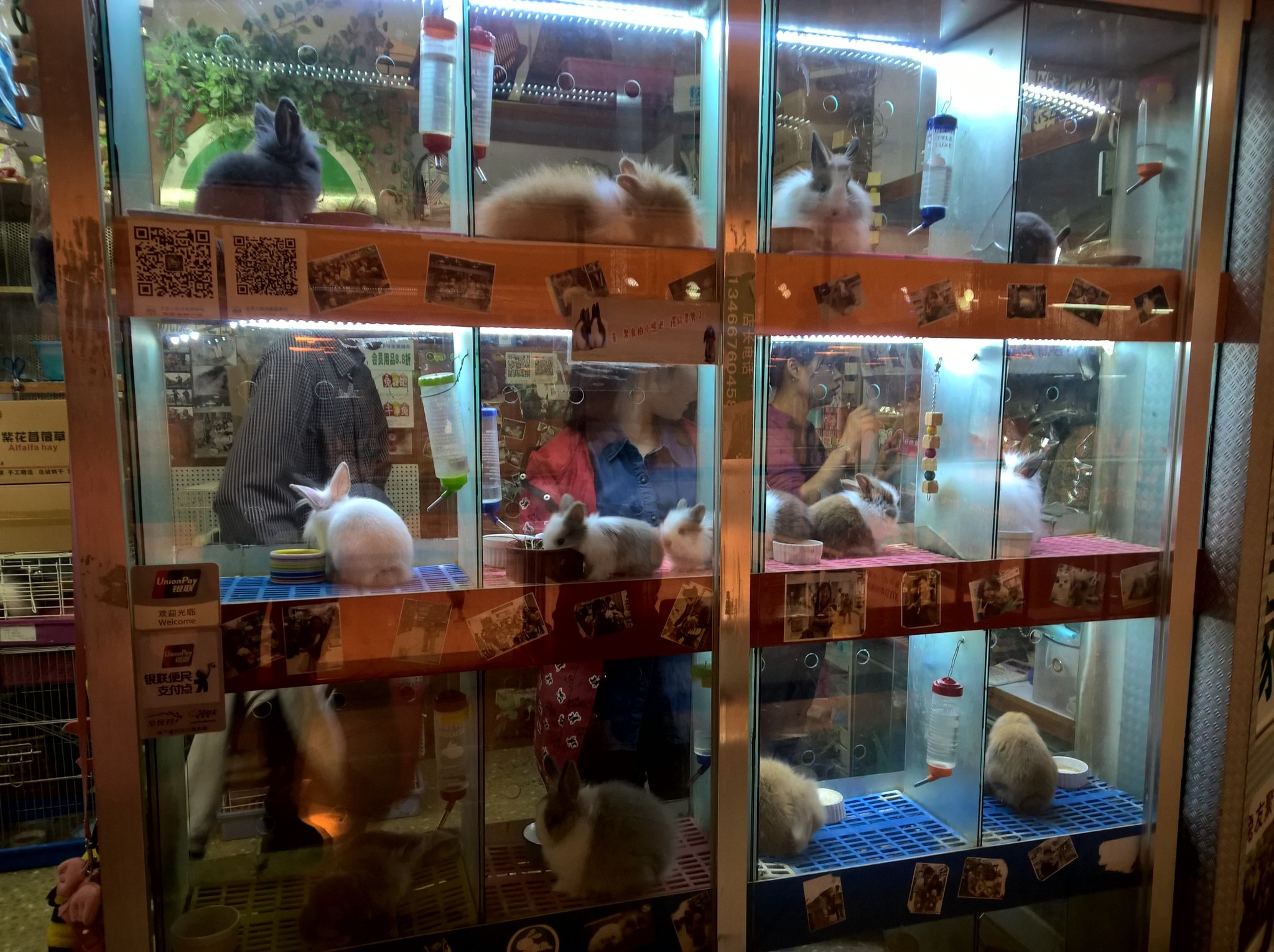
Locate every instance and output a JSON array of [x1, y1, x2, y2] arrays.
[[181, 321, 393, 876], [764, 332, 1092, 792], [513, 352, 713, 812]]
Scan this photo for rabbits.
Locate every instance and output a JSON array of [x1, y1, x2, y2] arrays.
[[703, 324, 717, 364], [825, 279, 861, 319], [918, 448, 1048, 561], [587, 910, 653, 952], [758, 754, 827, 861], [1011, 211, 1073, 265], [192, 96, 325, 224], [532, 753, 678, 899], [473, 155, 709, 243], [571, 301, 606, 351], [541, 493, 664, 580], [766, 488, 813, 562], [772, 133, 874, 255], [983, 711, 1057, 817], [298, 831, 466, 948], [660, 497, 716, 571], [289, 461, 416, 587], [807, 474, 901, 558]]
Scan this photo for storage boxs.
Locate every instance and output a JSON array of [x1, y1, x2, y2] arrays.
[[0, 398, 76, 485], [0, 483, 73, 554]]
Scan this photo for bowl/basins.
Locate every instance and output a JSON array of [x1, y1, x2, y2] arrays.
[[270, 548, 328, 584], [996, 522, 1036, 559], [817, 784, 846, 826], [1051, 754, 1090, 790], [483, 528, 585, 586], [1076, 251, 1144, 269], [306, 208, 379, 229], [772, 536, 824, 566], [521, 819, 561, 866], [171, 904, 242, 952]]
